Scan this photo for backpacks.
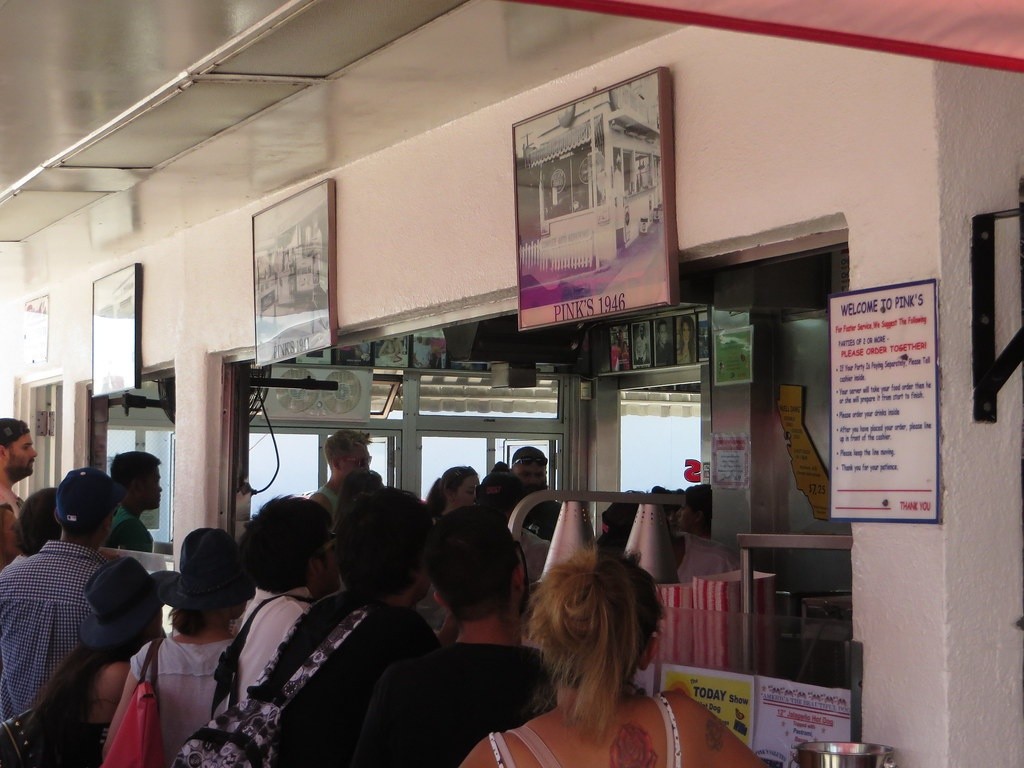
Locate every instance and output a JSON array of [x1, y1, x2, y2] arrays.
[[171, 595, 383, 768], [0, 706, 68, 768]]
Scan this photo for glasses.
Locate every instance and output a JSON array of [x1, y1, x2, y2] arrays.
[[345, 456, 370, 469], [314, 533, 338, 558], [513, 456, 547, 467]]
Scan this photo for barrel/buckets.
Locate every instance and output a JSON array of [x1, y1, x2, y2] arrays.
[[798, 741, 898, 768]]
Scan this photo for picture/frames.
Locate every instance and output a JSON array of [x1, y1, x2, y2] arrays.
[[251, 180, 337, 368], [91, 264, 142, 394], [510, 68, 678, 331]]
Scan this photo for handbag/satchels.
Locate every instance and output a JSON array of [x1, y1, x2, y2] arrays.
[[100, 637, 166, 768]]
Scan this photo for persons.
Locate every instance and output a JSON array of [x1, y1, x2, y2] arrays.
[[655, 320, 674, 365], [611, 328, 629, 372], [635, 324, 649, 364], [414, 337, 432, 368], [675, 316, 693, 364], [0, 416, 770, 768]]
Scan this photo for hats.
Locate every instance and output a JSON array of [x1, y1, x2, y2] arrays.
[[56, 467, 126, 524], [157, 526, 257, 609], [76, 556, 180, 649], [0, 418, 30, 445]]
